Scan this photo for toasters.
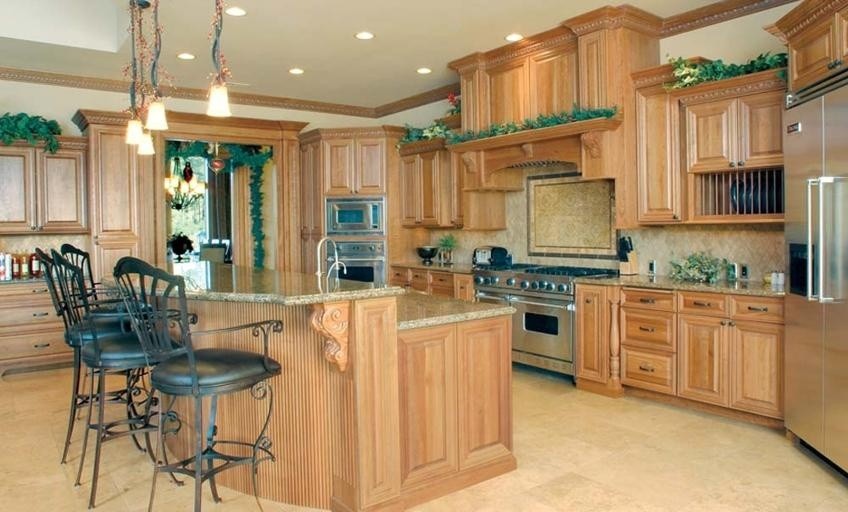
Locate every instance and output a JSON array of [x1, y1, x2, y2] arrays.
[[324, 196, 385, 238]]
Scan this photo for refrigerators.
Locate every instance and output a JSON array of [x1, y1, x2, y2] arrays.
[[783, 68, 848, 475]]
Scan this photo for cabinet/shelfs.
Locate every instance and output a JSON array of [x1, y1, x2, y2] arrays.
[[391, 262, 477, 303], [399, 138, 458, 231], [0, 279, 74, 375], [762, 0, 848, 95], [301, 126, 407, 286], [447, 4, 660, 229], [574, 273, 786, 432], [630, 56, 783, 226], [0, 134, 91, 236], [70, 109, 141, 312]]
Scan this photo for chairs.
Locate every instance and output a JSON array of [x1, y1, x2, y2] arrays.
[[35, 247, 153, 465], [51, 249, 185, 509], [60, 243, 136, 420], [112, 257, 283, 512], [221, 239, 230, 256], [211, 239, 219, 244], [199, 244, 225, 264]]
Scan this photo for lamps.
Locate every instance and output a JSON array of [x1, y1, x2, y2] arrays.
[[206, 0, 233, 118], [137, 1, 155, 156], [165, 157, 205, 212], [125, 0, 145, 145], [145, 0, 169, 132]]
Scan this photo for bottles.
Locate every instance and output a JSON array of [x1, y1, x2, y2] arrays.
[[40, 250, 48, 279], [30, 247, 40, 278], [1, 254, 4, 279], [12, 250, 20, 278], [21, 249, 29, 278], [5, 252, 11, 278]]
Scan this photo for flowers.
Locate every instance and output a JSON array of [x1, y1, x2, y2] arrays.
[[436, 233, 456, 250]]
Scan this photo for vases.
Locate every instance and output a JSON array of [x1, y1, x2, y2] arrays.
[[438, 250, 454, 264]]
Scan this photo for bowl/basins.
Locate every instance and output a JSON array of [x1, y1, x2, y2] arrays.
[[416, 246, 438, 261]]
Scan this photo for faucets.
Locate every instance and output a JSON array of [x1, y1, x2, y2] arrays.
[[326, 261, 347, 279], [315, 236, 340, 279]]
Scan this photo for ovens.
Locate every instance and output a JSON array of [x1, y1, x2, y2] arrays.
[[325, 242, 385, 285], [477, 287, 573, 363]]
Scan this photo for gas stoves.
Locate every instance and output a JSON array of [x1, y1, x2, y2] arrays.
[[473, 264, 619, 295]]
